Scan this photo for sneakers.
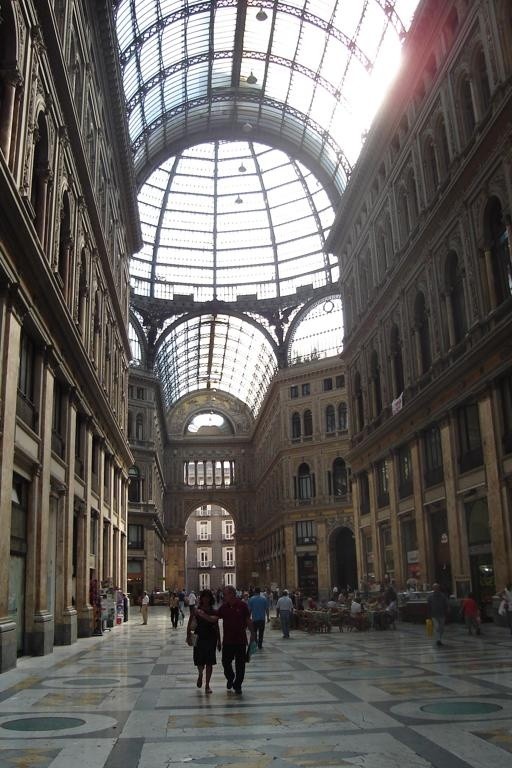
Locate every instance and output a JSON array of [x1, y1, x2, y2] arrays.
[[197, 674, 243, 694], [436, 640, 443, 646]]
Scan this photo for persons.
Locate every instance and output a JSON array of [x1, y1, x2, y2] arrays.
[[462, 594, 482, 636], [427, 583, 449, 648], [123, 594, 129, 622], [141, 591, 149, 624], [194, 585, 256, 694], [138, 594, 144, 612], [246, 586, 270, 649], [167, 583, 398, 638], [185, 588, 221, 694], [502, 586, 512, 635]]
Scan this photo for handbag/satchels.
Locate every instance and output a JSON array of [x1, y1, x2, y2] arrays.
[[190, 618, 198, 630], [426, 619, 433, 636]]
